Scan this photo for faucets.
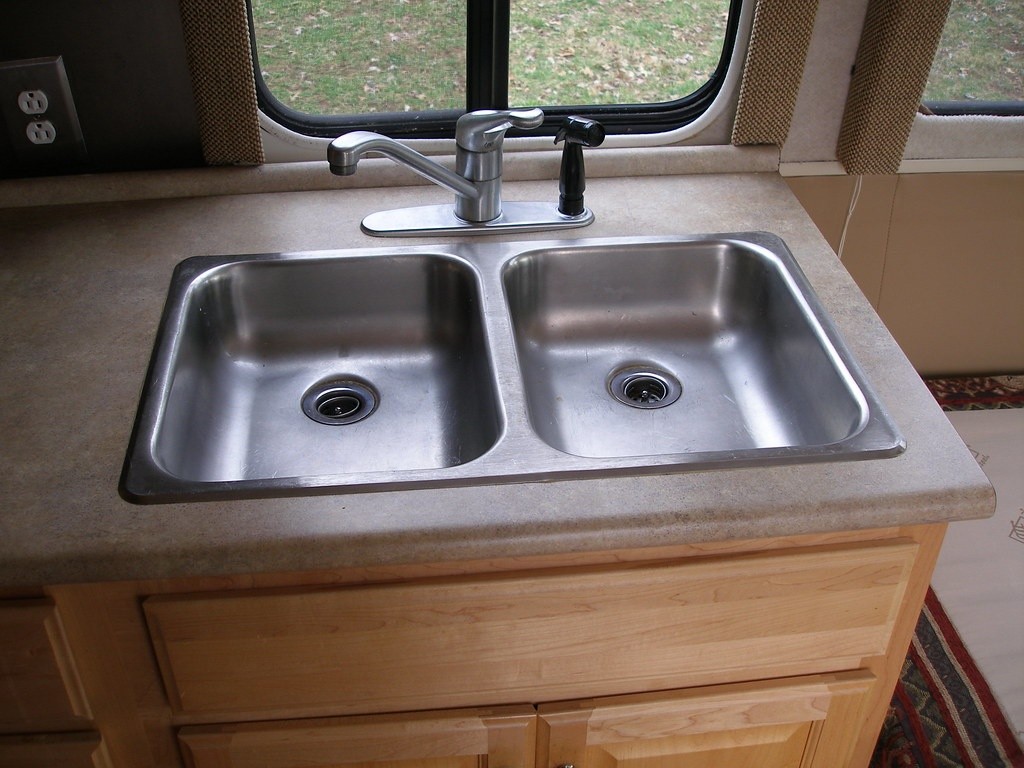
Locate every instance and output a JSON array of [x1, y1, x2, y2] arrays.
[[326, 107, 548, 224]]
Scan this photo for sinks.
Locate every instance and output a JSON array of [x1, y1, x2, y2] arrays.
[[116, 242, 510, 506], [489, 230, 909, 481]]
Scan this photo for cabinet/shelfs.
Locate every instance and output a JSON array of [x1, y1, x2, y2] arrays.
[[0, 522, 952, 768]]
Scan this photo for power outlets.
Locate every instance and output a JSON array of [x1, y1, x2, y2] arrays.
[[0, 56, 80, 150]]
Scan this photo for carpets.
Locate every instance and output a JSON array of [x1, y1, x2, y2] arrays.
[[865, 371, 1024, 768]]
